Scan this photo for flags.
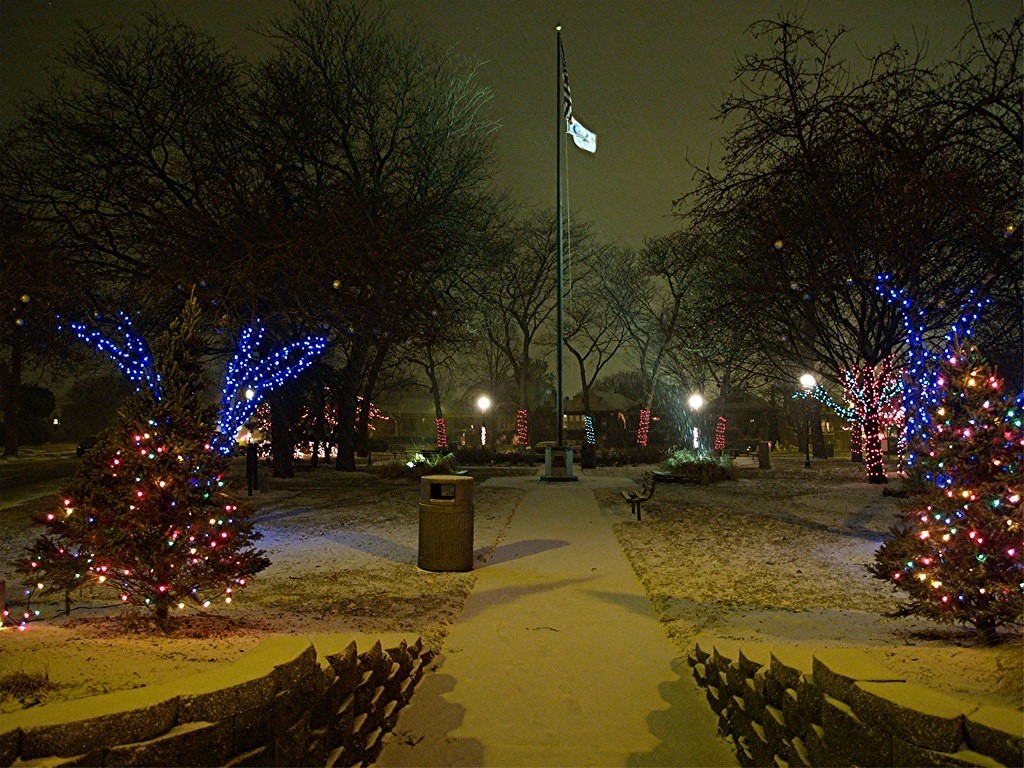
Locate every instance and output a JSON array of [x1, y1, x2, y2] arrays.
[[560, 41, 572, 122], [567, 116, 597, 153]]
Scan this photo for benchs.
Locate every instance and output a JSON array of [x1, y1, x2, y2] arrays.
[[750, 446, 759, 460], [725, 449, 740, 461], [619, 470, 655, 521]]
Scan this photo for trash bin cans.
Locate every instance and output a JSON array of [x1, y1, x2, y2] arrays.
[[824, 444, 834, 457], [417, 474, 475, 572], [581, 444, 597, 470]]
